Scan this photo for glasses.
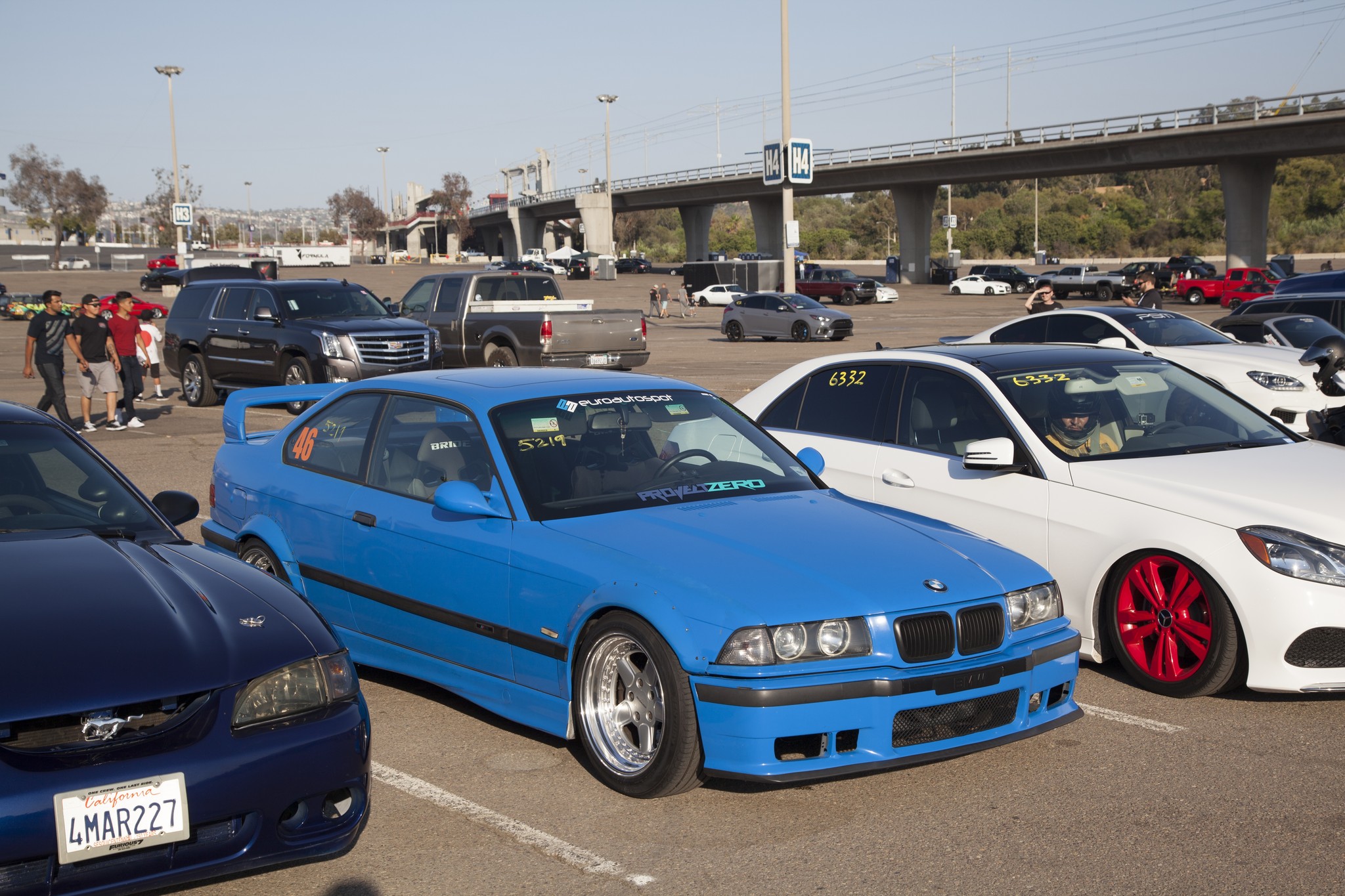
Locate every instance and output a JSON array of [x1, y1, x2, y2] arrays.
[[1139, 280, 1149, 286], [1040, 289, 1053, 295], [86, 303, 102, 307]]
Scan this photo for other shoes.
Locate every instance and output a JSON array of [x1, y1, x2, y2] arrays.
[[650, 313, 696, 318], [156, 394, 169, 401], [135, 395, 143, 402]]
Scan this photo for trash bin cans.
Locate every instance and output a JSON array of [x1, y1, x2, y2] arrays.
[[371, 255, 385, 264], [1047, 257, 1060, 265], [1271, 254, 1294, 275], [932, 269, 958, 286], [931, 259, 946, 268], [567, 266, 590, 279]]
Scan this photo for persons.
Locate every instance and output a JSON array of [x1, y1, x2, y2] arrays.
[[689, 295, 698, 317], [799, 263, 806, 279], [649, 284, 664, 318], [659, 283, 672, 318], [677, 283, 689, 318], [105, 291, 152, 428], [1170, 269, 1178, 288], [70, 294, 127, 431], [1045, 376, 1118, 457], [22, 290, 89, 434], [1122, 272, 1162, 310], [1025, 284, 1064, 314], [135, 309, 170, 401], [547, 258, 553, 263]]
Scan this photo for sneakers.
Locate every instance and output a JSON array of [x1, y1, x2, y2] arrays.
[[127, 416, 145, 428], [105, 421, 127, 430], [82, 421, 97, 432], [115, 407, 123, 423], [71, 427, 81, 434]]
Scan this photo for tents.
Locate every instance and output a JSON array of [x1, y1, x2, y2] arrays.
[[568, 251, 603, 276], [546, 246, 581, 266]]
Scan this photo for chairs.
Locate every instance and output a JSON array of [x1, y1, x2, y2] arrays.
[[824, 272, 832, 282], [572, 411, 680, 499], [912, 398, 979, 459], [408, 426, 493, 518], [1040, 379, 1123, 450]]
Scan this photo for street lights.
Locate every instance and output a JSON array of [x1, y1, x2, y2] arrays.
[[377, 147, 392, 264], [596, 93, 620, 256], [243, 181, 253, 245], [154, 65, 189, 270]]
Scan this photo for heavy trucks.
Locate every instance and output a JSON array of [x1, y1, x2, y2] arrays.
[[256, 246, 351, 267]]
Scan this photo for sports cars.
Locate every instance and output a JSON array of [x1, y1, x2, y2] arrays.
[[199, 364, 1086, 798]]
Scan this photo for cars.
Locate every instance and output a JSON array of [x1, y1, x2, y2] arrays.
[[460, 248, 487, 258], [189, 240, 212, 250], [484, 248, 588, 276], [1031, 253, 1345, 361], [147, 253, 179, 271], [936, 305, 1345, 444], [0, 398, 373, 896], [241, 253, 260, 258], [949, 274, 1011, 295], [691, 284, 758, 307], [139, 266, 178, 292], [868, 279, 898, 304], [50, 257, 91, 270], [665, 341, 1345, 698], [615, 258, 653, 274]]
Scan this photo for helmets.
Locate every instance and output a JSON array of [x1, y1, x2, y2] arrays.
[[1046, 376, 1102, 448], [1299, 335, 1345, 397]]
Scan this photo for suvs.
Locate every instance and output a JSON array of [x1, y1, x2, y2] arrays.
[[721, 291, 855, 342], [969, 264, 1040, 294], [158, 263, 445, 409]]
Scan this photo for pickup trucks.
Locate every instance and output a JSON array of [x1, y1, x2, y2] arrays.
[[779, 268, 878, 305], [384, 268, 651, 374]]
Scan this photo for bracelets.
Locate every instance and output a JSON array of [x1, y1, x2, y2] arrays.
[[659, 300, 660, 301]]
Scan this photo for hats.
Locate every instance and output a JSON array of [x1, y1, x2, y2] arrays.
[[141, 309, 155, 320], [81, 293, 97, 308], [654, 285, 659, 288]]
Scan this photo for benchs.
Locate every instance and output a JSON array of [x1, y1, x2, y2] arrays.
[[307, 426, 505, 494]]
